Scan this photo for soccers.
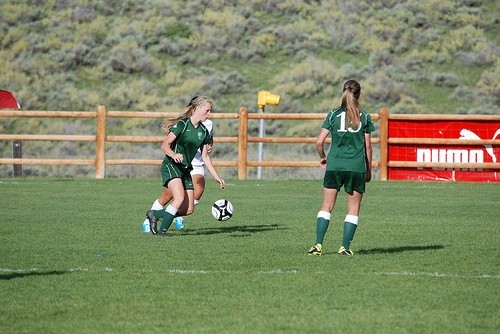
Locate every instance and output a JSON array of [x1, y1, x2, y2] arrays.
[[212, 199, 234, 221]]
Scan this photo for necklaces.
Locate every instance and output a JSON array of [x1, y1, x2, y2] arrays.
[[192, 122, 199, 125]]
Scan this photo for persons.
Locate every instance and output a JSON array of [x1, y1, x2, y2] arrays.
[[306, 79, 375, 256], [147, 96, 225, 236], [142, 95, 214, 233]]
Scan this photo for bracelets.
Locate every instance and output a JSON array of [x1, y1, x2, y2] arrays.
[[217, 177, 223, 183]]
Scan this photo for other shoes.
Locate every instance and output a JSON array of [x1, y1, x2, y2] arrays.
[[175, 217, 185, 230], [308, 244, 322, 256], [338, 246, 354, 256], [143, 219, 150, 233]]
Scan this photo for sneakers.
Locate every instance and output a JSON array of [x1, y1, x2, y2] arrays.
[[147, 210, 157, 234]]
